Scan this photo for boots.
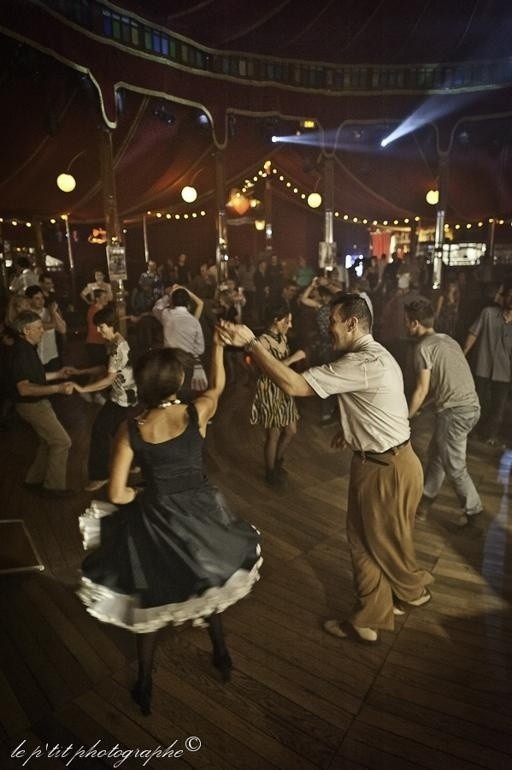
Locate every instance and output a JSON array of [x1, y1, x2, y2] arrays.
[[412, 494, 434, 521]]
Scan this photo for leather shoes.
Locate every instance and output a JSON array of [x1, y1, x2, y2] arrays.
[[324, 617, 378, 643], [393, 588, 431, 616]]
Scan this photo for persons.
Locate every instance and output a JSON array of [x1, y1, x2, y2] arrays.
[[0, 251, 512, 717]]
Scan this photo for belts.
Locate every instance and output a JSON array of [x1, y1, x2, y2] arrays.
[[354, 440, 408, 466]]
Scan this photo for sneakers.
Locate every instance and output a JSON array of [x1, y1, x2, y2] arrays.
[[84, 479, 109, 492]]
